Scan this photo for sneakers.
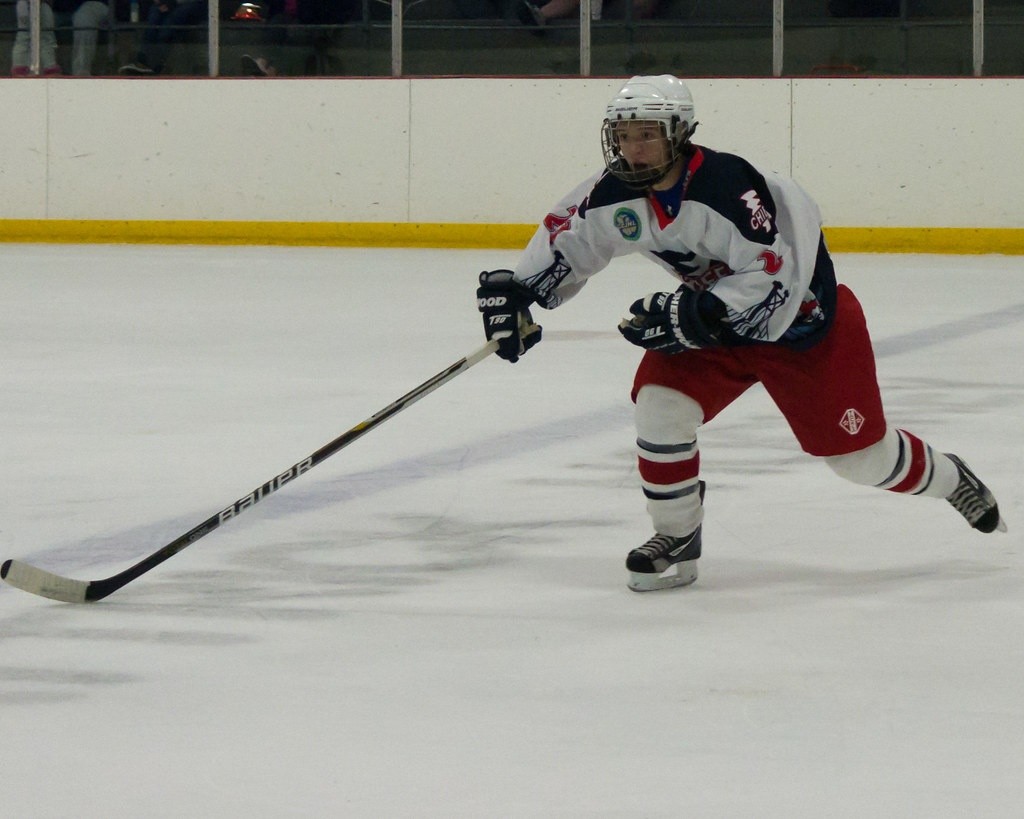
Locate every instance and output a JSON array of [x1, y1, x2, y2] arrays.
[[626, 527, 702, 593], [942, 452, 1008, 534]]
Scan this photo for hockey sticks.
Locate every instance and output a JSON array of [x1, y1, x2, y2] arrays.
[[2, 320, 533, 603]]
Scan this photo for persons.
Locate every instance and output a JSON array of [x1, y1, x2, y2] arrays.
[[1, 0, 1023, 75], [516, 72, 1009, 595]]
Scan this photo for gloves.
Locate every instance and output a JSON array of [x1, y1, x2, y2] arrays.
[[476, 269, 542, 363], [617, 291, 731, 357]]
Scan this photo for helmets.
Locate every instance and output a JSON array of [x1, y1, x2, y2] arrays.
[[604, 74, 698, 156]]
